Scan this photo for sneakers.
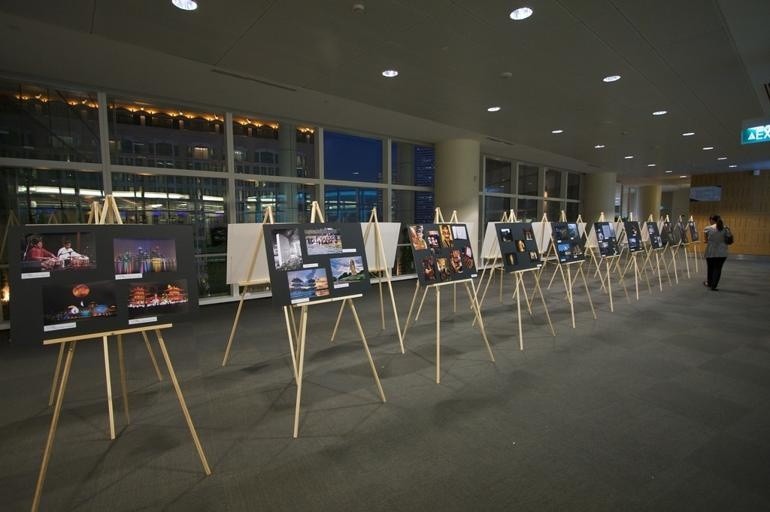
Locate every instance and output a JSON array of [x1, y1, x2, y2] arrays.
[[703, 281, 720, 292]]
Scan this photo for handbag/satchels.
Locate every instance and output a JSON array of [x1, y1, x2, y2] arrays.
[[724, 226, 734, 245]]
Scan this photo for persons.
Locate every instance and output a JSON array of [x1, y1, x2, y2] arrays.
[[58, 241, 80, 256], [410, 225, 462, 280], [27, 238, 56, 270], [703, 214, 734, 290], [318, 232, 337, 245]]
